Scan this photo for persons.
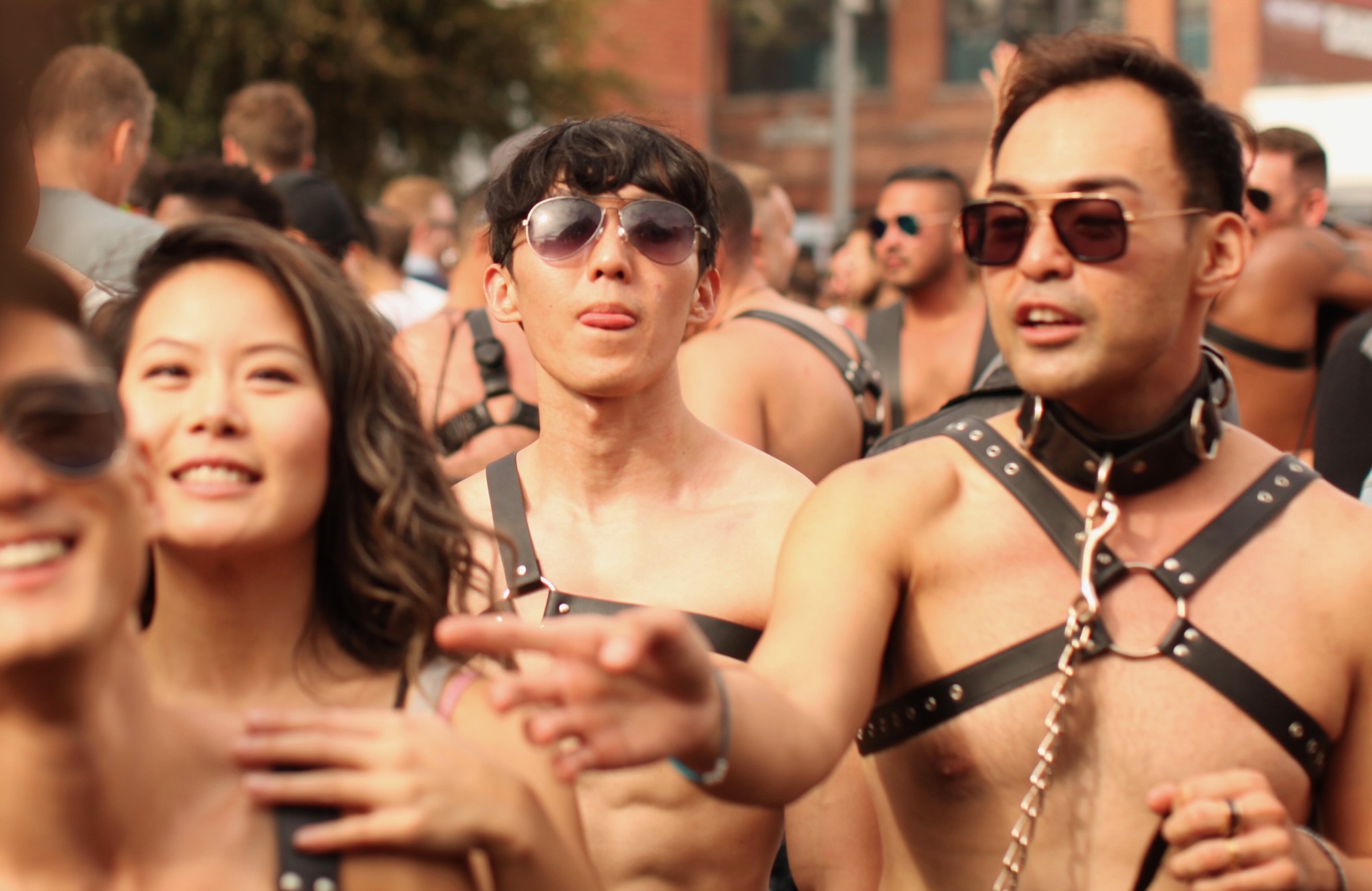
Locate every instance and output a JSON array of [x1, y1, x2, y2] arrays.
[[0, 44, 1372, 502], [0, 217, 598, 891], [449, 115, 878, 891], [434, 28, 1372, 891]]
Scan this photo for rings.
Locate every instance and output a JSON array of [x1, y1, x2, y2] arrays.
[[1228, 800, 1236, 836]]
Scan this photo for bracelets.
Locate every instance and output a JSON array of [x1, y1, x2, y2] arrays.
[[1293, 828, 1347, 891], [670, 669, 730, 785]]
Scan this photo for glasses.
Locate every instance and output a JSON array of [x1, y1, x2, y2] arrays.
[[1, 373, 128, 473], [1240, 187, 1312, 212], [868, 211, 961, 240], [507, 195, 711, 266], [959, 191, 1221, 267]]
